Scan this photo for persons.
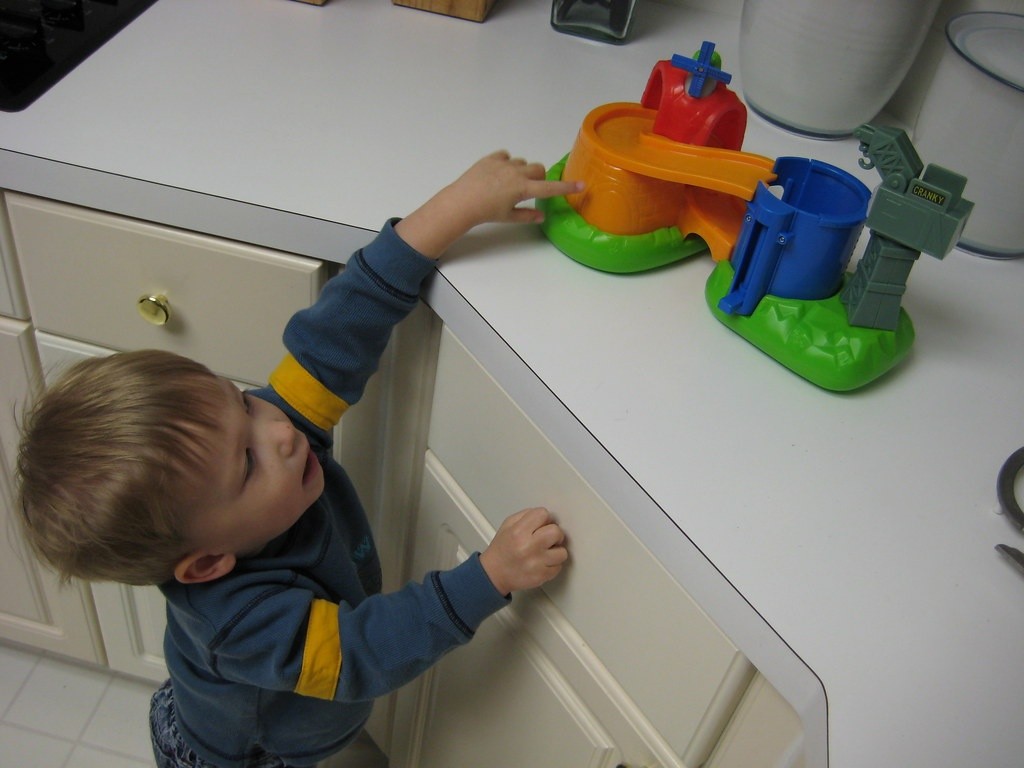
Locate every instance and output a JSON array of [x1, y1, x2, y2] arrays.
[[14, 149, 585, 768]]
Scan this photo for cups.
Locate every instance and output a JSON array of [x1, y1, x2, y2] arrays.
[[913, 12, 1024, 259], [739, 0, 945, 139]]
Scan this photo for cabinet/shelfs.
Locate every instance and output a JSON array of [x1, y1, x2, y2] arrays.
[[387, 319, 758, 768], [0, 187, 322, 682]]
